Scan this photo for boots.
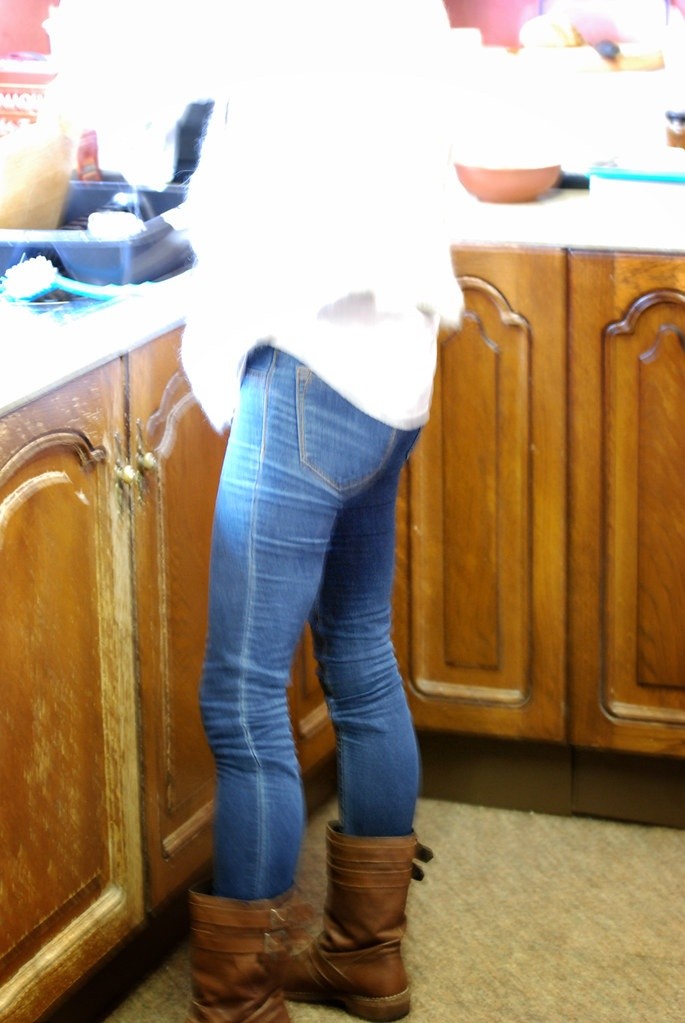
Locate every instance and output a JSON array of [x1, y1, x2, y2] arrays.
[[278, 818, 435, 1021], [181, 880, 302, 1023]]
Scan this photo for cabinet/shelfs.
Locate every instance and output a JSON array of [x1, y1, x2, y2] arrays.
[[304, 240, 683, 831], [0, 322, 302, 1023]]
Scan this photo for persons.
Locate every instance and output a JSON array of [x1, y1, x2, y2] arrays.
[[181, 0, 466, 1023]]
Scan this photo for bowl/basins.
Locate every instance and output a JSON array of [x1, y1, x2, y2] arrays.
[[454, 163, 561, 205]]
